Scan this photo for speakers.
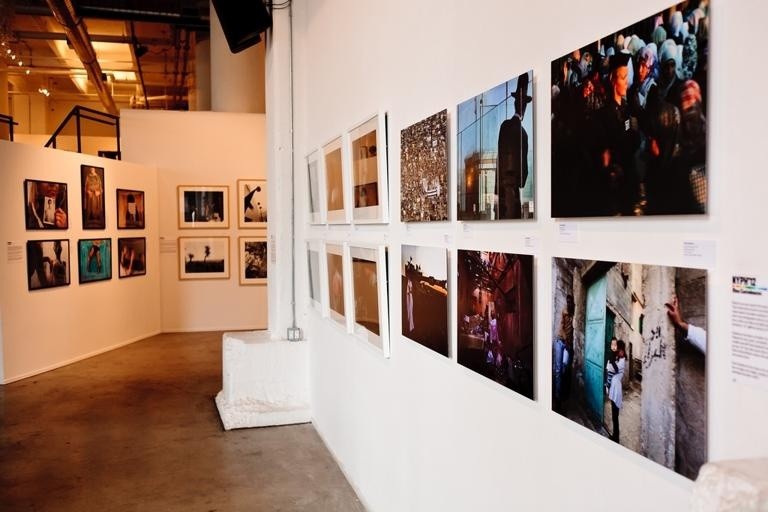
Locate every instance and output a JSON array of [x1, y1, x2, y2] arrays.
[[212, 0, 272, 53]]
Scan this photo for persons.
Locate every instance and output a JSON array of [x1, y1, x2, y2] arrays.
[[405, 269, 415, 338], [84, 167, 103, 225], [119, 245, 145, 276], [607, 340, 628, 443], [665, 296, 706, 356], [27, 181, 67, 228], [552, 0, 711, 217], [45, 200, 56, 223], [552, 331, 574, 399], [87, 240, 102, 273], [27, 242, 66, 287], [603, 337, 619, 398], [493, 73, 532, 220]]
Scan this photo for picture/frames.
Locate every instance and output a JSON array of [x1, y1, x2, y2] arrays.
[[77, 164, 113, 284], [320, 109, 393, 359], [116, 187, 147, 278], [176, 176, 267, 287], [23, 179, 71, 292]]
